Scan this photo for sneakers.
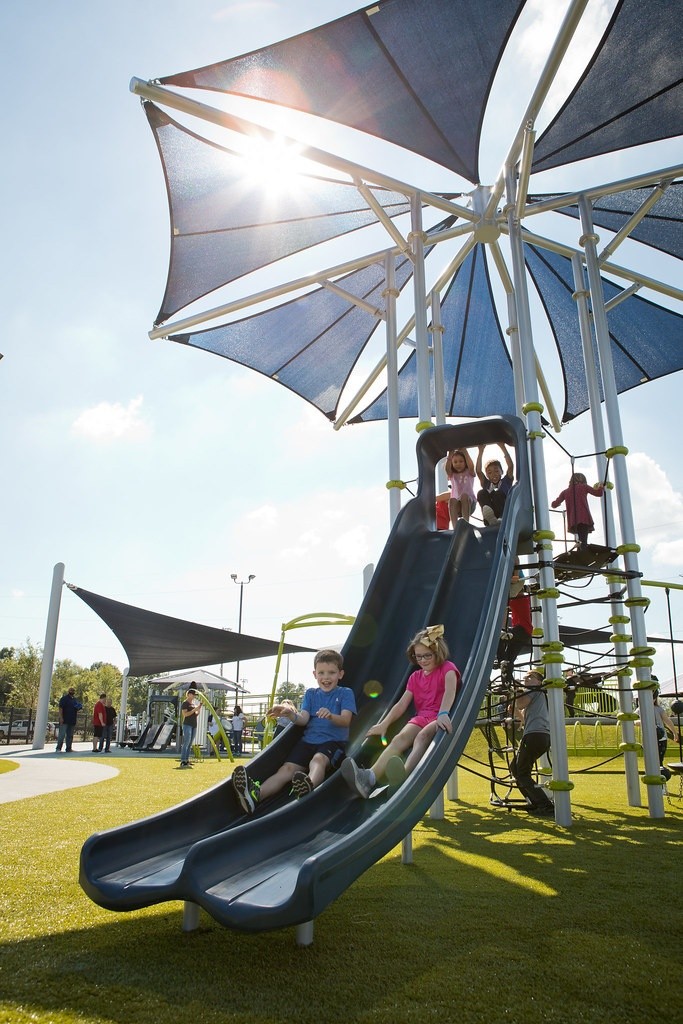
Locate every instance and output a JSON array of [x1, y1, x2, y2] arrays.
[[288, 771, 312, 800], [92, 749, 99, 752], [384, 755, 404, 800], [104, 748, 112, 752], [342, 757, 371, 799], [231, 764, 260, 815], [528, 806, 554, 815], [179, 761, 193, 768]]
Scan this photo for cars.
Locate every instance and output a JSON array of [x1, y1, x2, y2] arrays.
[[0, 719, 66, 741], [114, 716, 142, 736]]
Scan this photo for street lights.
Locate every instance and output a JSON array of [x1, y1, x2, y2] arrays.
[[241, 678, 248, 715], [230, 573, 256, 717]]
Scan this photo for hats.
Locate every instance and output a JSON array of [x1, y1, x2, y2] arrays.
[[188, 689, 198, 695]]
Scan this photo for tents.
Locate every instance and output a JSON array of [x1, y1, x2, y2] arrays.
[[146, 668, 251, 754], [126, 0, 683, 827]]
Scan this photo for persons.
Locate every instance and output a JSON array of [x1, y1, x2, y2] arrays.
[[179, 688, 204, 769], [230, 649, 358, 815], [91, 693, 107, 753], [340, 623, 462, 803], [444, 446, 476, 531], [98, 698, 118, 753], [496, 694, 525, 748], [635, 666, 659, 720], [562, 667, 577, 718], [508, 554, 526, 599], [475, 441, 514, 527], [551, 472, 605, 552], [507, 670, 556, 816], [55, 687, 82, 752], [207, 703, 266, 758], [632, 689, 679, 771], [492, 586, 533, 695], [272, 699, 299, 740]]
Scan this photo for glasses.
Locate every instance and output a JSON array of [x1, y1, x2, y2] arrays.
[[409, 651, 434, 661], [521, 674, 538, 679]]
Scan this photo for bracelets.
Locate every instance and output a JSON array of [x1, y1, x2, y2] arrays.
[[437, 711, 449, 719], [504, 454, 510, 458], [291, 713, 298, 723], [446, 457, 452, 461], [673, 733, 678, 736]]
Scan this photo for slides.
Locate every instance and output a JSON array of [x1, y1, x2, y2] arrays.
[[131, 694, 179, 752], [75, 412, 538, 950]]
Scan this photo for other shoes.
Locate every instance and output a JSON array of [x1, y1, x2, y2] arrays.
[[66, 749, 74, 752], [55, 748, 61, 753], [490, 687, 509, 693]]
